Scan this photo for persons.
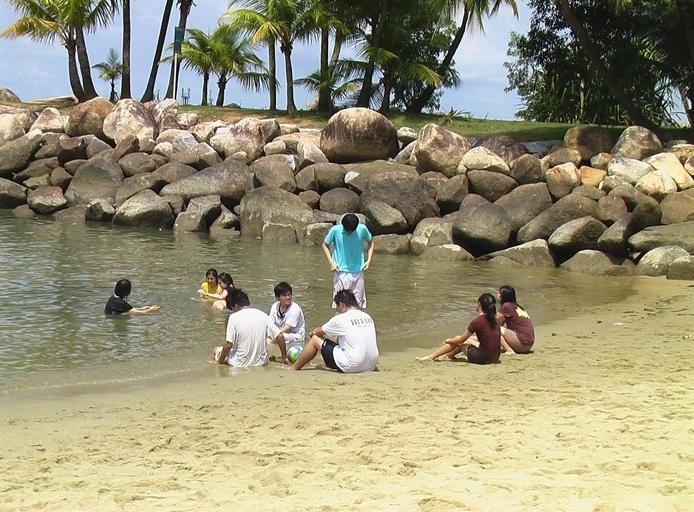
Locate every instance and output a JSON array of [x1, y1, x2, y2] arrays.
[[321, 213, 375, 315], [192, 268, 222, 303], [288, 289, 379, 374], [415, 293, 500, 364], [105, 279, 160, 317], [198, 272, 240, 311], [213, 288, 291, 367], [266, 281, 305, 346], [496, 285, 535, 355]]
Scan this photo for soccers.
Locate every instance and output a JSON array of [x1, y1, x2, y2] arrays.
[[288, 346, 304, 363]]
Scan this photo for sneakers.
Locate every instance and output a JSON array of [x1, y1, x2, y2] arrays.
[[435, 354, 452, 361], [415, 355, 435, 364]]
